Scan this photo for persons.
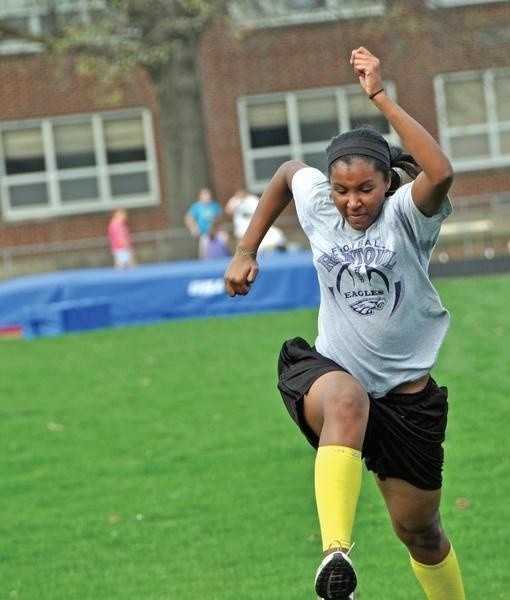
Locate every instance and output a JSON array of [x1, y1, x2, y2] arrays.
[[184, 185, 286, 258], [107, 204, 140, 268], [218, 44, 477, 600]]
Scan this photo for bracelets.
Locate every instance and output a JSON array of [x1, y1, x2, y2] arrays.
[[367, 87, 385, 100]]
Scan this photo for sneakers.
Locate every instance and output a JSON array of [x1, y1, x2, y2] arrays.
[[314, 541, 358, 600]]
